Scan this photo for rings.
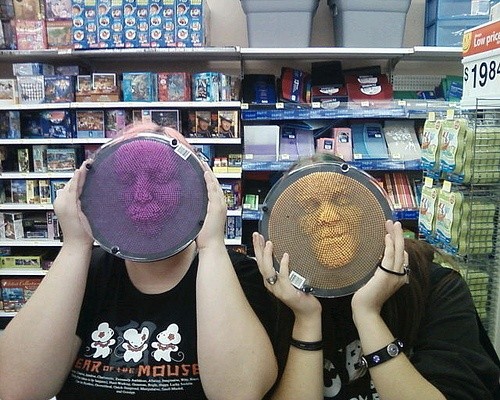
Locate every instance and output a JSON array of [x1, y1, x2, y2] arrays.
[[406, 265, 410, 275], [266, 276, 277, 285]]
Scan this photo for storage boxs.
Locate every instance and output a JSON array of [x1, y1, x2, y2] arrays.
[[424, 0, 500, 47], [0, 0, 240, 314], [327, 0, 411, 48], [240, 0, 319, 49]]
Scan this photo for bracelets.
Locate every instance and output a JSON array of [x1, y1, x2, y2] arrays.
[[378, 261, 406, 276], [290, 338, 324, 351], [361, 338, 404, 368]]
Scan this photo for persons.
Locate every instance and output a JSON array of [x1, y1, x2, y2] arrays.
[[250, 153, 500, 400], [0, 123, 277, 400]]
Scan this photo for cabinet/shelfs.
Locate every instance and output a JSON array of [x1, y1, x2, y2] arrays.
[[0, 46, 241, 329], [241, 45, 465, 259]]
